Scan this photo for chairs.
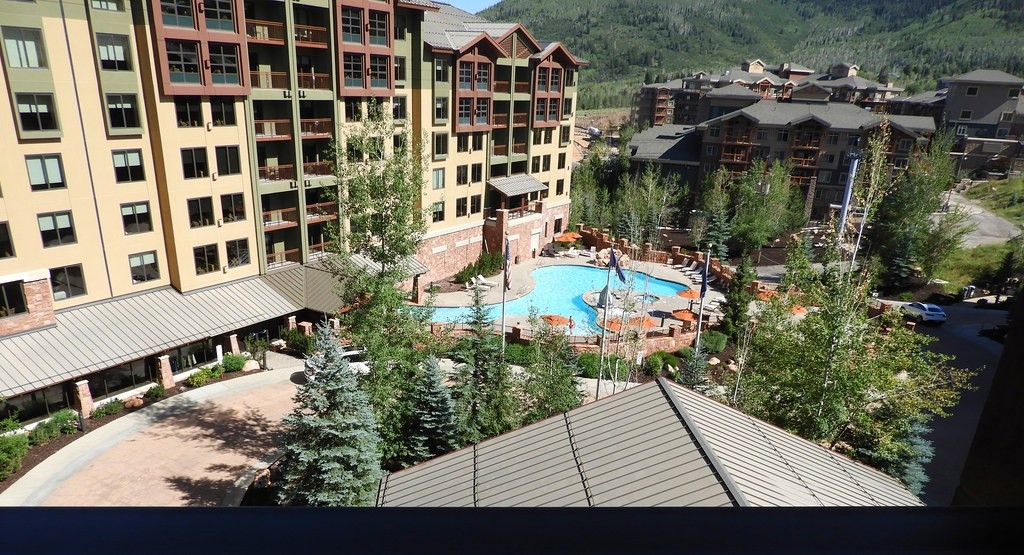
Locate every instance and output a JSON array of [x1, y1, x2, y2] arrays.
[[554, 246, 599, 258], [703, 298, 726, 313], [465, 274, 499, 294], [667, 257, 717, 285]]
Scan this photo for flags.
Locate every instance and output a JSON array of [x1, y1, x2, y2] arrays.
[[504, 239, 511, 291], [699, 252, 707, 299], [610, 247, 626, 284]]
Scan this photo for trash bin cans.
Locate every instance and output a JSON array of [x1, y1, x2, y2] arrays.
[[515, 256, 520, 264], [962, 287, 968, 299], [968, 286, 976, 298]]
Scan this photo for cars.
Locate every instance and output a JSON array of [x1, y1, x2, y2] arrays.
[[899, 302, 948, 326]]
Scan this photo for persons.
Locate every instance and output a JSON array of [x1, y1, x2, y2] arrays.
[[569, 316, 575, 336]]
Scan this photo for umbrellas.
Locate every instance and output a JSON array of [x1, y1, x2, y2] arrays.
[[540, 315, 569, 326], [600, 318, 628, 331], [630, 317, 658, 328], [676, 289, 700, 309], [673, 308, 699, 321], [553, 233, 583, 242], [757, 291, 780, 301]]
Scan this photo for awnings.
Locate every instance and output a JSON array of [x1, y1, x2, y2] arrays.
[[487, 173, 548, 197]]
